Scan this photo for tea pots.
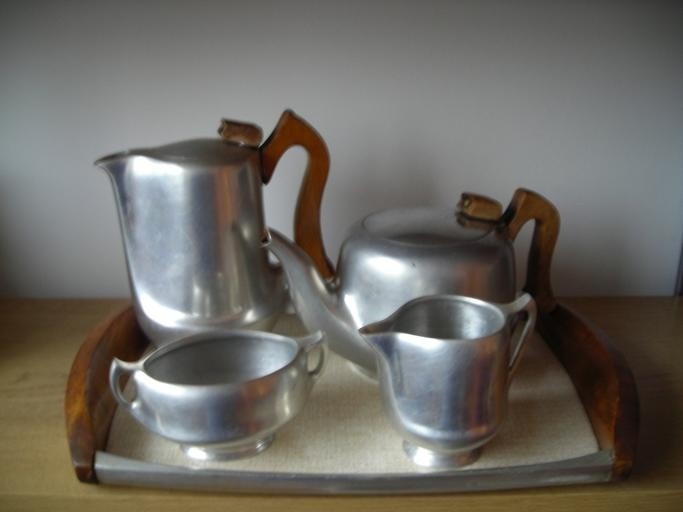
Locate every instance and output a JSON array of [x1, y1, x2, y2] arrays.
[[351, 290, 540, 471], [258, 184, 562, 390], [92, 106, 335, 351]]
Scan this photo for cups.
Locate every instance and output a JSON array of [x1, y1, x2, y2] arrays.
[[101, 327, 330, 466]]
